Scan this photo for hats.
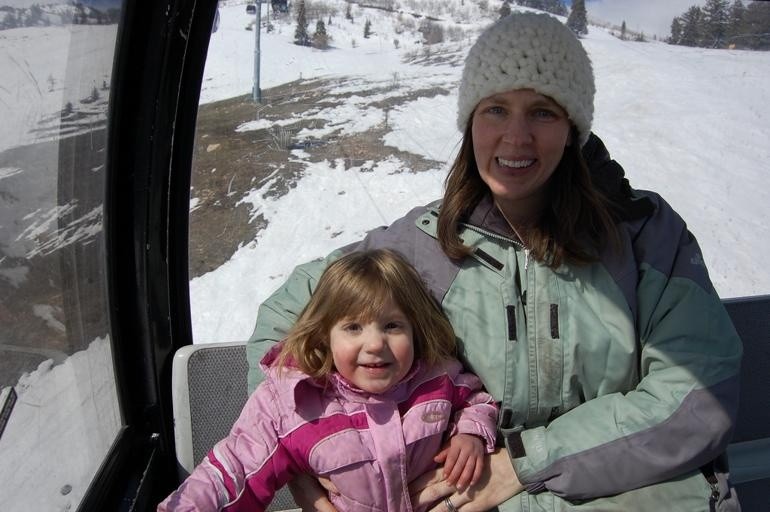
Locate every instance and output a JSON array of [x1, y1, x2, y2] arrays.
[[458, 13, 595, 149]]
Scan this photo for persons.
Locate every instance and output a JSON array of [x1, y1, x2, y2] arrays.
[[245, 11, 745, 512], [157, 246, 500, 512]]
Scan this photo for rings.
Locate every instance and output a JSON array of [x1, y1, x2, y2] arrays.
[[444, 497, 455, 511]]
